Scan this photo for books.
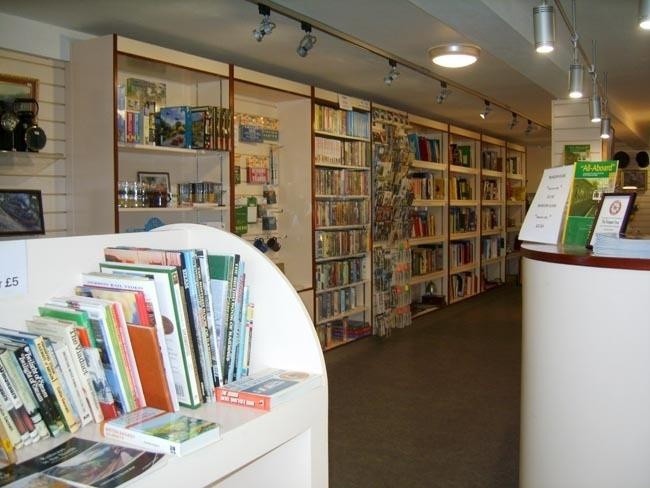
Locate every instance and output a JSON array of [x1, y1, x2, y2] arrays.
[[406, 133, 443, 277], [449, 143, 472, 168], [450, 206, 478, 300], [448, 173, 472, 200], [481, 206, 506, 290], [507, 156, 522, 201], [117, 78, 232, 152], [507, 206, 523, 276], [1, 246, 323, 487], [315, 103, 370, 350], [177, 181, 222, 206], [233, 112, 279, 233], [481, 149, 503, 200]]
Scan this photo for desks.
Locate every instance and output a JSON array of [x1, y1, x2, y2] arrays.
[[2, 223, 330, 486]]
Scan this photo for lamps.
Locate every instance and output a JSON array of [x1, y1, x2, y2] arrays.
[[428, 44, 482, 69], [252, 3, 533, 137], [636, 0, 650, 31], [532, 0, 610, 140]]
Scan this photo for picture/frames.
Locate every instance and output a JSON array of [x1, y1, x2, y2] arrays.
[[1, 72, 38, 134], [136, 169, 170, 194], [1, 189, 45, 238], [619, 170, 647, 192]]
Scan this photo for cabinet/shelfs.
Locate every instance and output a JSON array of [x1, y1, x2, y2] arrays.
[[312, 86, 374, 353], [405, 113, 529, 321], [234, 67, 316, 324], [65, 37, 230, 238]]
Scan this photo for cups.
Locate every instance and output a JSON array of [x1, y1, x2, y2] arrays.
[[267, 236, 281, 251], [150, 183, 172, 207], [253, 237, 268, 253], [117, 181, 150, 207]]
